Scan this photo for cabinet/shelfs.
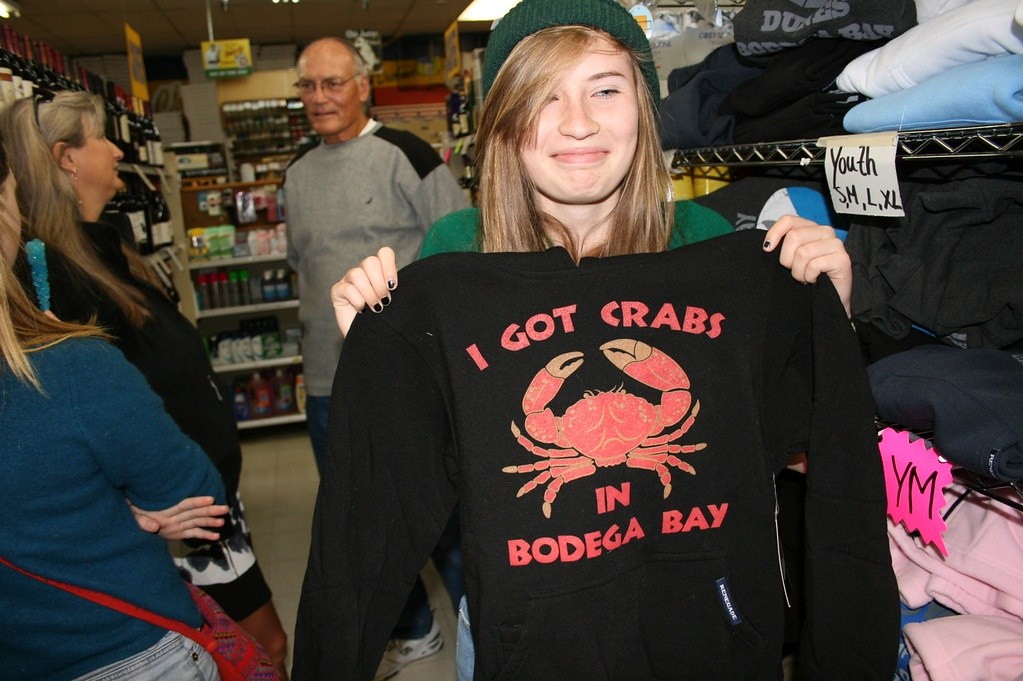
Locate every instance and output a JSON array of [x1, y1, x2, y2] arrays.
[[118, 161, 183, 272], [669, 124, 1023, 513], [188, 249, 309, 433]]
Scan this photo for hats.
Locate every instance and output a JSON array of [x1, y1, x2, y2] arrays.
[[482, 0, 660, 122]]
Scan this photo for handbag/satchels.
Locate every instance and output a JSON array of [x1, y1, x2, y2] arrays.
[[185, 582, 283, 680]]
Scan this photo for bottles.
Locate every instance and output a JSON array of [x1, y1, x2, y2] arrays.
[[0, 18, 176, 259], [232, 368, 305, 422], [197, 264, 292, 309]]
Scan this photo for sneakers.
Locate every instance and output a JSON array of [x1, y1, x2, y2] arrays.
[[374, 621, 443, 681]]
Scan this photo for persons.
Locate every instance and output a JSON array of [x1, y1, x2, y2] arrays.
[[0, 145, 228, 681], [282, 38, 470, 681], [0, 91, 289, 681], [327, 0, 854, 681]]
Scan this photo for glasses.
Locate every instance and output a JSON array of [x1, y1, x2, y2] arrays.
[[32, 86, 55, 126], [293, 73, 360, 98]]
